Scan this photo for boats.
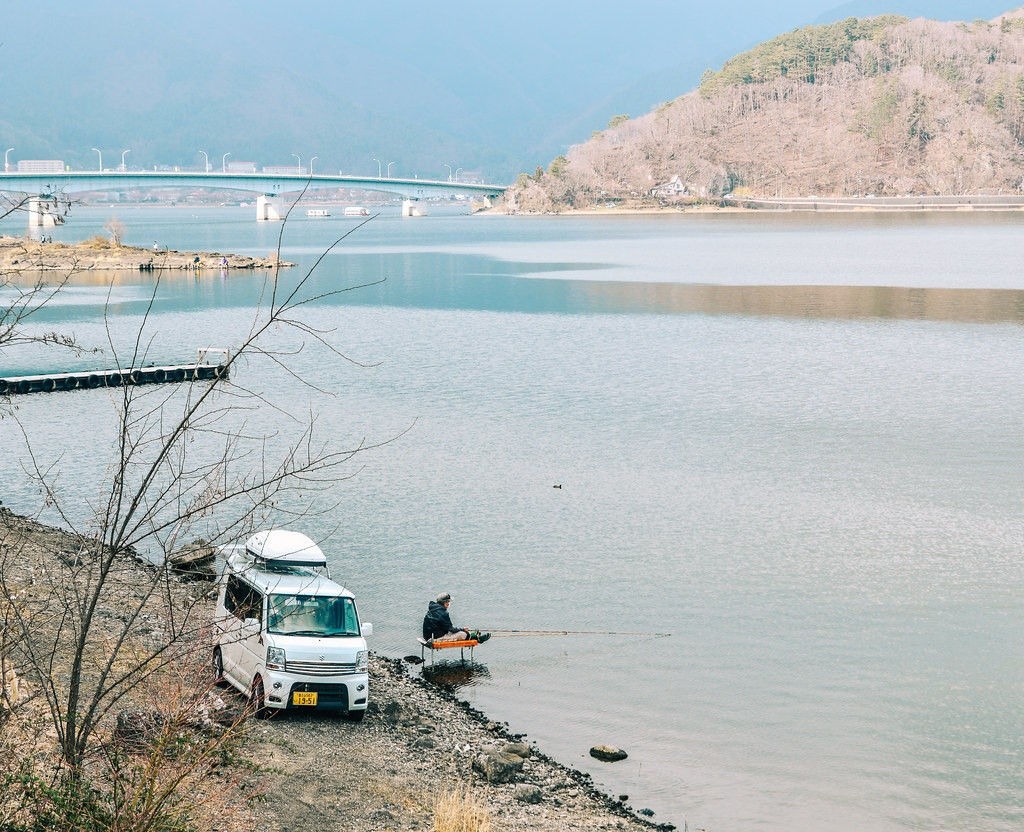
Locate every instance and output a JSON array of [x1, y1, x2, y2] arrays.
[[305, 209, 331, 216], [344, 206, 371, 216]]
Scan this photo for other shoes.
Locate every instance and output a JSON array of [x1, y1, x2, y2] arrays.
[[471, 631, 492, 644]]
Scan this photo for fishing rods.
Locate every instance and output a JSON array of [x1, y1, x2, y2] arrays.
[[468, 629, 677, 637]]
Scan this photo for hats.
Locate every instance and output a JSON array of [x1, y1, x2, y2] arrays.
[[436, 593, 454, 602]]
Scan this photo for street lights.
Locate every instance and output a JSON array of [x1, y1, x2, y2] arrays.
[[199, 151, 208, 174], [445, 165, 452, 182], [291, 154, 300, 175], [456, 168, 463, 183], [4, 148, 14, 172], [223, 152, 231, 173], [373, 159, 380, 178], [122, 150, 132, 172], [388, 162, 395, 179], [93, 149, 102, 172], [310, 156, 318, 177]]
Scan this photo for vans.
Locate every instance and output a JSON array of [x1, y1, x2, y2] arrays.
[[210, 529, 372, 723]]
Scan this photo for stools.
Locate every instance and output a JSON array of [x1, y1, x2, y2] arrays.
[[418, 637, 478, 673]]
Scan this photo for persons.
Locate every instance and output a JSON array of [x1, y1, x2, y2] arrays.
[[422, 592, 492, 644]]
